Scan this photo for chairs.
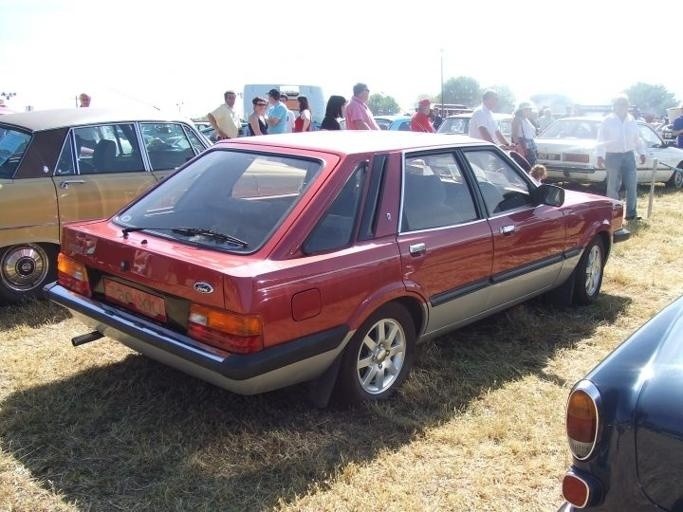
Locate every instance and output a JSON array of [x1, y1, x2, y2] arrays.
[[402, 174, 463, 229], [90, 139, 119, 171]]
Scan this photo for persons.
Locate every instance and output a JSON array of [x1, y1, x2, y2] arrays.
[[209, 90, 242, 141], [0, 99, 10, 139], [75, 93, 106, 158]]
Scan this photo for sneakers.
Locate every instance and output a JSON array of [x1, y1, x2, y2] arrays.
[[626, 216, 642, 220]]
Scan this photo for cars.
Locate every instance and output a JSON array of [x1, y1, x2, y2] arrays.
[[39, 125, 632, 421], [0, 106, 219, 302], [555, 290, 682, 512]]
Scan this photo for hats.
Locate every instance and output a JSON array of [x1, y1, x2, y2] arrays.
[[419, 100, 432, 107], [516, 102, 534, 111], [265, 89, 280, 100]]
